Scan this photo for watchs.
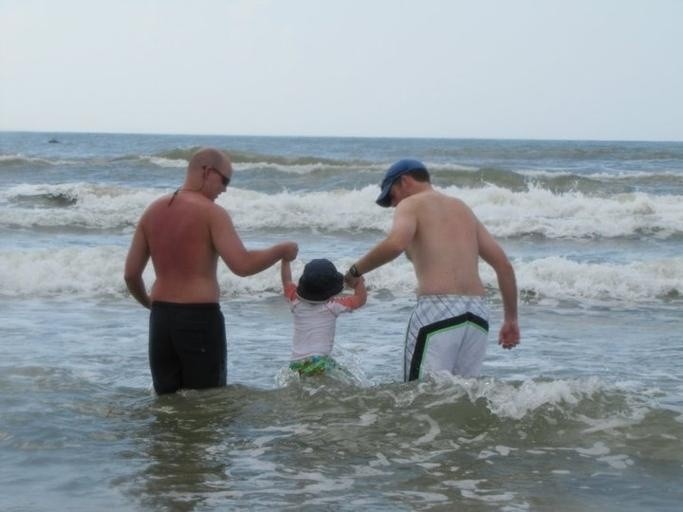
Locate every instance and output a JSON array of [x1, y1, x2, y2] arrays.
[[350, 265, 361, 277]]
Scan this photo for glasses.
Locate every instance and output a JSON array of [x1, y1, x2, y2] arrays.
[[202, 165, 230, 185]]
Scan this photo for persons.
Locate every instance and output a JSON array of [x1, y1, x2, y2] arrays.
[[123, 147, 298, 396], [281, 260, 367, 378], [345, 159, 520, 384]]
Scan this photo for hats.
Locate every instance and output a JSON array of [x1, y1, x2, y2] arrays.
[[294, 258, 344, 304], [375, 158, 429, 208]]
